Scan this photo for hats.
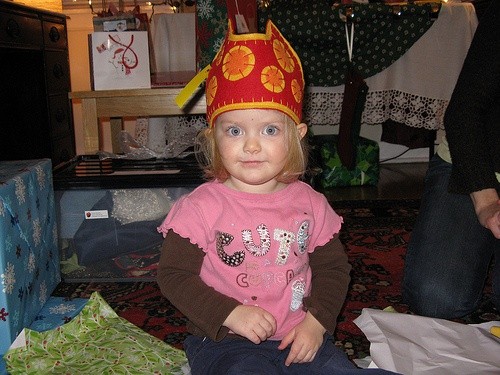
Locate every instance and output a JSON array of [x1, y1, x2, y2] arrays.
[[205, 19, 305, 126]]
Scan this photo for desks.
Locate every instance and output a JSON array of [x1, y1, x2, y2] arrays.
[[68, 87, 208, 155], [201, 0, 476, 161]]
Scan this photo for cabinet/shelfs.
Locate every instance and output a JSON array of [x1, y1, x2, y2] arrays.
[[0, 0, 77, 181]]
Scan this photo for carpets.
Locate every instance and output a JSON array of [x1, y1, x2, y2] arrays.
[[51, 198, 500, 369]]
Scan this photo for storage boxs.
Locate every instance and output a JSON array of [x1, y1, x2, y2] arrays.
[[0, 158, 62, 354], [310, 135, 379, 188]]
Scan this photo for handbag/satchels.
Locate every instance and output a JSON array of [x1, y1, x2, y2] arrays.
[[150, 2, 196, 88], [89, 31, 151, 91], [120, 0, 150, 41], [92, 0, 136, 31]]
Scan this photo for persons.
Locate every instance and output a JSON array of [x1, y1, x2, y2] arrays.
[[400, 0, 500, 323], [156, 18, 368, 375]]
